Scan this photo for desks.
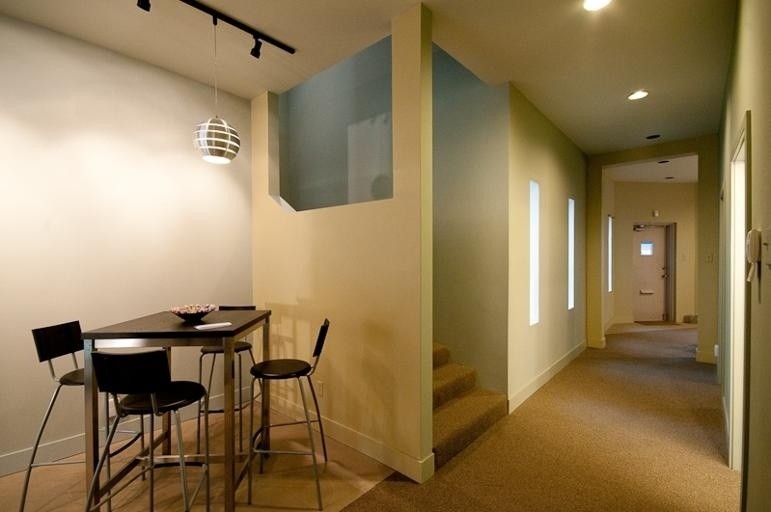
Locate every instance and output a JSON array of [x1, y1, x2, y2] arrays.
[[78, 305, 273, 511]]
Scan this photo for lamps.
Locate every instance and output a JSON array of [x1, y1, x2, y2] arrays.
[[193, 13, 239, 164], [136, 0, 152, 12], [250, 38, 262, 61]]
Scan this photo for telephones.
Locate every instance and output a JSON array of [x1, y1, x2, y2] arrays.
[[746, 228, 759, 263]]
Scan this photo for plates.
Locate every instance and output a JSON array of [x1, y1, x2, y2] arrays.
[[171, 304, 215, 322]]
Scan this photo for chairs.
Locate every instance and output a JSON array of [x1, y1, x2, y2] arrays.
[[23, 320, 148, 511], [196, 305, 264, 459], [80, 345, 215, 508], [246, 316, 327, 510]]
